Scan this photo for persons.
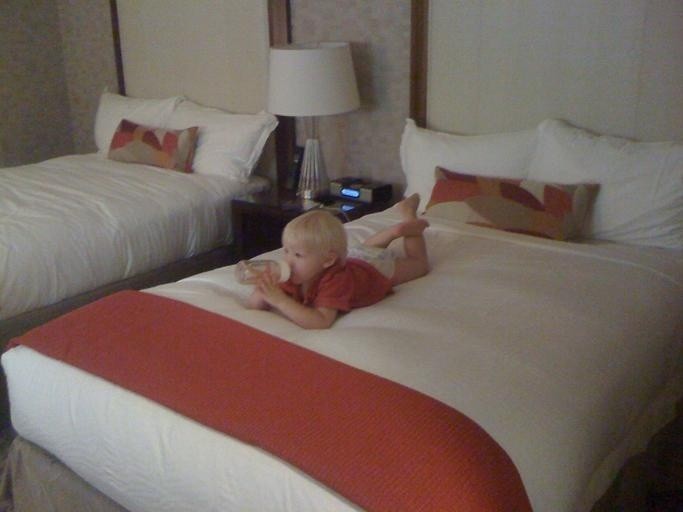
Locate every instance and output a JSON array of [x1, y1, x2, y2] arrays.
[[245, 193, 430, 330]]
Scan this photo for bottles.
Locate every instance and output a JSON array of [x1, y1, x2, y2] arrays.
[[235, 257, 289, 285]]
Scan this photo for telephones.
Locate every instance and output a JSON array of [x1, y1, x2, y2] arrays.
[[286, 147, 303, 190]]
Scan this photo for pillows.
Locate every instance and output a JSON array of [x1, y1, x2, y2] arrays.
[[408, 155, 602, 248], [397, 111, 574, 217], [85, 84, 284, 185], [511, 114, 683, 253]]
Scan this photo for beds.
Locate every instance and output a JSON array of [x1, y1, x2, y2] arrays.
[[0, 85, 275, 433], [0, 117, 681, 512]]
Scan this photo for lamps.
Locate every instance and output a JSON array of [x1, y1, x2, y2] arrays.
[[261, 38, 365, 204]]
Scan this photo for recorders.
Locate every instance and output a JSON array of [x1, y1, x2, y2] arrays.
[[331, 175, 392, 204]]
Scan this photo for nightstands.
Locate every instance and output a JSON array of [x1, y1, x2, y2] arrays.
[[229, 182, 396, 266]]
